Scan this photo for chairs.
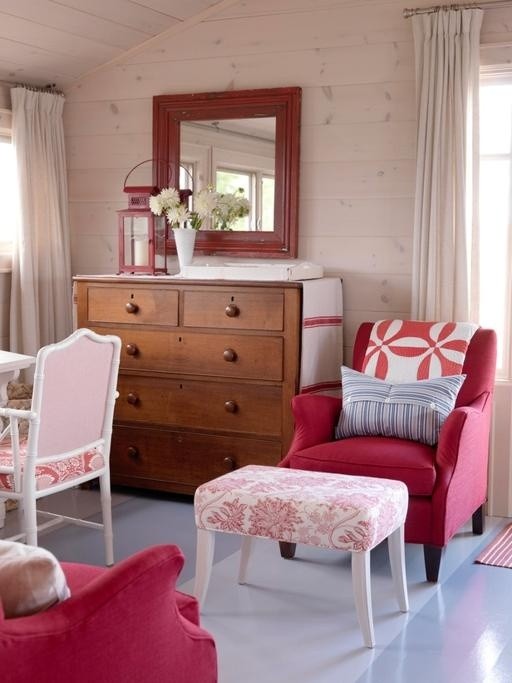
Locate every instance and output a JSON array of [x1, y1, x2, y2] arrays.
[[0, 544, 218, 683], [0, 328, 122, 567], [278, 322, 497, 583]]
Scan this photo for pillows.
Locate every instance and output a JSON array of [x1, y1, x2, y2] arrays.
[[335, 319, 480, 446], [0, 539, 71, 619]]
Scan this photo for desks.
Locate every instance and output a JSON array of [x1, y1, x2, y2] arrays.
[[0, 349, 33, 407]]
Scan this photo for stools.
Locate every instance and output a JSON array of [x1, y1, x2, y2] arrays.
[[193, 464, 409, 648]]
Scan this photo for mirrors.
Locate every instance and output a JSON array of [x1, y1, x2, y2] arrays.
[[150, 87, 302, 260]]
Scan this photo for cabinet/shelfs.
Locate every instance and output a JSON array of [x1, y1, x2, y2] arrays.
[[72, 277, 299, 496]]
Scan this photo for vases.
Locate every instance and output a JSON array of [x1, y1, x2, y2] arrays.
[[171, 229, 196, 278]]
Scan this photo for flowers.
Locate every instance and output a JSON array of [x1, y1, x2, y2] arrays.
[[195, 184, 252, 232], [148, 187, 218, 228]]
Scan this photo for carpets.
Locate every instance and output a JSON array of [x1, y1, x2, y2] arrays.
[[475, 522, 512, 569]]
